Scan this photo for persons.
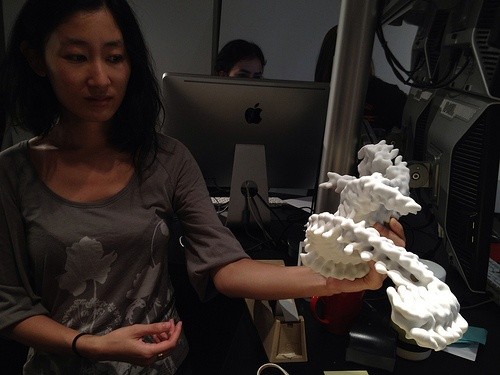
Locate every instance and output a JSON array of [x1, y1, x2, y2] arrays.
[[214, 38, 268, 81], [313, 24, 410, 134], [0, 0, 410, 375]]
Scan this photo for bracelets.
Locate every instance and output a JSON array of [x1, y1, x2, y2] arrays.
[[72, 332, 97, 366]]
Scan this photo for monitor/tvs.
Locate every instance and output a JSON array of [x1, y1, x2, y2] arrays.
[[406, 0, 499, 98], [401, 82, 499, 293], [161, 73, 328, 198]]
